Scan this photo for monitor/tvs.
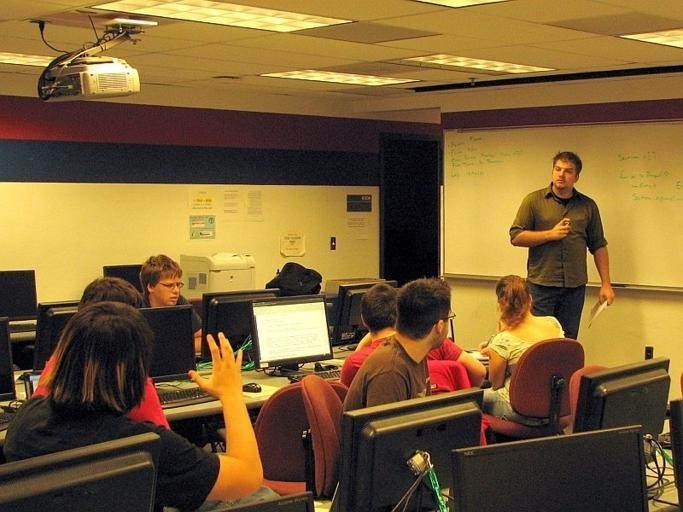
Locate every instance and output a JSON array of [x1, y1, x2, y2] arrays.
[[669, 398, 683, 512], [202, 288, 282, 363], [2, 430, 162, 512], [34, 300, 81, 370], [203, 491, 315, 512], [338, 280, 398, 345], [1, 269, 38, 318], [134, 303, 197, 382], [449, 425, 650, 512], [103, 264, 146, 294], [247, 293, 334, 376], [334, 386, 485, 511], [1, 316, 17, 403], [573, 355, 670, 467], [46, 306, 81, 370]]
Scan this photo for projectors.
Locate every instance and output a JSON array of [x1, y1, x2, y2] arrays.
[[37, 50, 142, 103]]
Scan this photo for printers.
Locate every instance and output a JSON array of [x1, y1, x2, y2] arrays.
[[179, 250, 256, 299]]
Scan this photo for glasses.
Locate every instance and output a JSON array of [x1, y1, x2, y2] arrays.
[[437, 310, 456, 321], [160, 282, 184, 288]]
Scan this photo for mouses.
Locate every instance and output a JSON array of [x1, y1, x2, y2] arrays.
[[242, 381, 262, 393]]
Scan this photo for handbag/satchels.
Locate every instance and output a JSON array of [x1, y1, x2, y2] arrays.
[[265, 262, 322, 296]]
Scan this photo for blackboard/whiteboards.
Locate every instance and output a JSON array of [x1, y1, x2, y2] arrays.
[[438, 118, 682, 295]]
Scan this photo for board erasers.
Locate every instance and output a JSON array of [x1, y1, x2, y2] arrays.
[[610, 284, 628, 287]]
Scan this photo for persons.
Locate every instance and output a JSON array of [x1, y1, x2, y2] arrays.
[[477, 274, 567, 428], [31, 276, 173, 435], [140, 253, 204, 353], [342, 273, 451, 414], [339, 281, 486, 388], [508, 150, 617, 342], [1, 301, 265, 510]]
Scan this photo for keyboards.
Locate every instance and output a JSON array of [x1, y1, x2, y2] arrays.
[[1, 411, 18, 431], [287, 369, 339, 383], [157, 386, 220, 410], [659, 432, 673, 449]]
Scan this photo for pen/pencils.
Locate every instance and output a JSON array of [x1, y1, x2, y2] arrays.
[[485, 336, 494, 348]]
